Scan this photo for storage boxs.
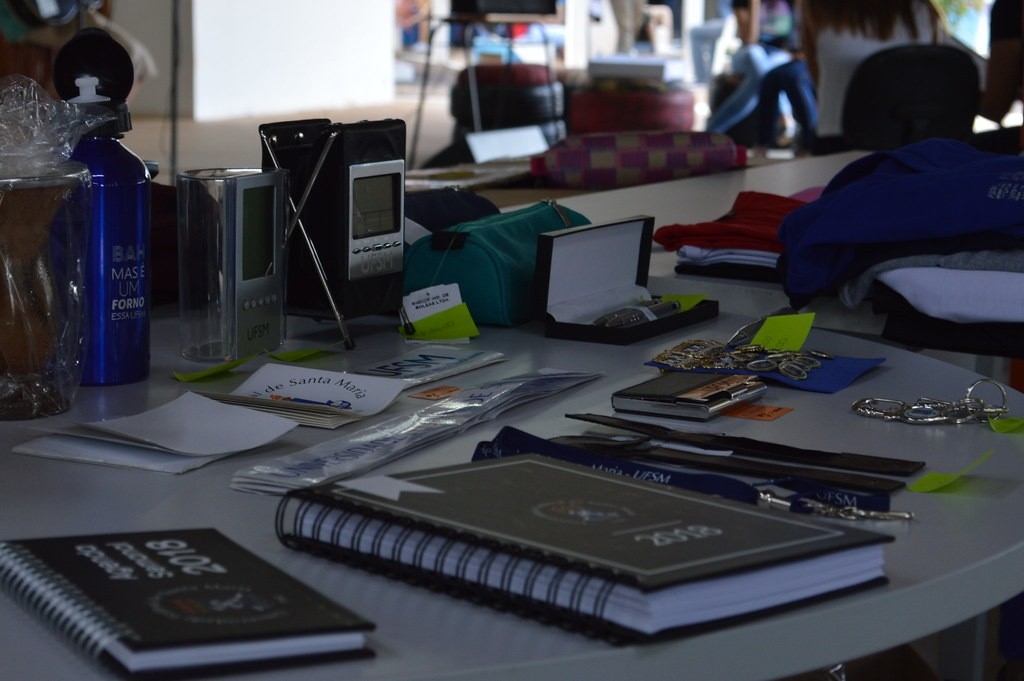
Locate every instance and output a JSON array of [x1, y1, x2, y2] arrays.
[[533, 215, 719, 344]]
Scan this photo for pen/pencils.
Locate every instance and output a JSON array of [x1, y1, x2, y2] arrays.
[[592, 299, 680, 328]]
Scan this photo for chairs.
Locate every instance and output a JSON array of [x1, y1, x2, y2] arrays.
[[808, 44, 982, 157]]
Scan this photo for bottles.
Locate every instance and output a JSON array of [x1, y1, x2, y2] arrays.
[[52, 26, 154, 388]]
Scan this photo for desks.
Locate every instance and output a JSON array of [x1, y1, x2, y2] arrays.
[[0, 276, 1024, 681]]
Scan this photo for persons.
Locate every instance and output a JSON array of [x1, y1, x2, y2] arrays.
[[705, 0, 1024, 158]]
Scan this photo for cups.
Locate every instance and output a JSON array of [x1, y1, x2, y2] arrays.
[[175, 166, 293, 372], [0, 162, 91, 423]]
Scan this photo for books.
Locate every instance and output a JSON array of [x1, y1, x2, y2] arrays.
[[274, 451, 896, 647], [0, 527, 379, 681]]
